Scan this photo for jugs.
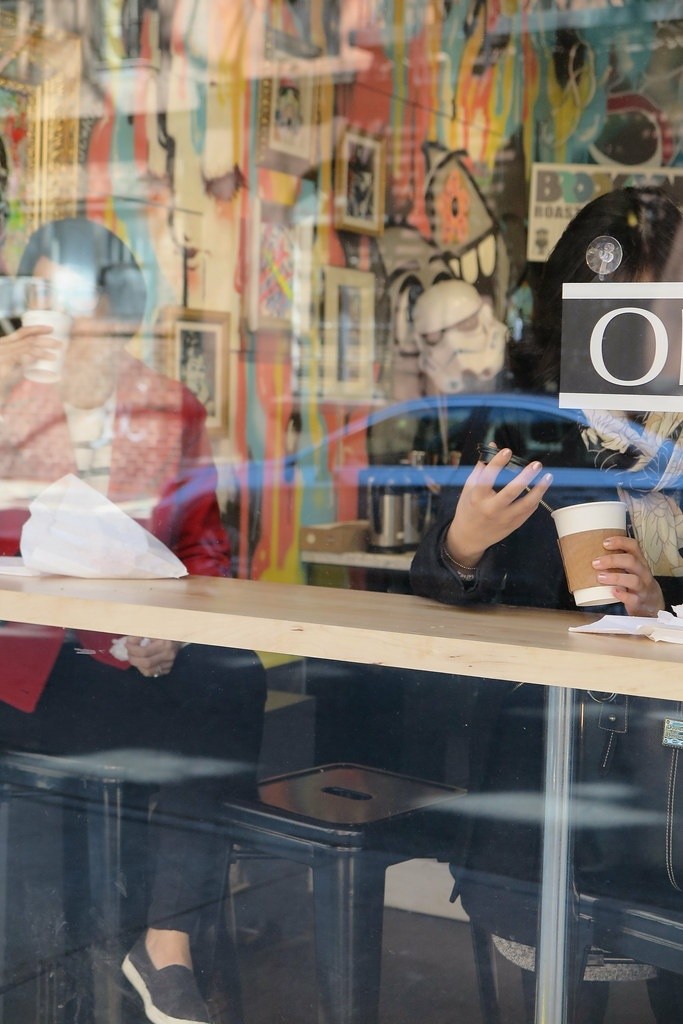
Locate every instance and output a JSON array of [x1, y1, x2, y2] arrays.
[[362, 470, 430, 553]]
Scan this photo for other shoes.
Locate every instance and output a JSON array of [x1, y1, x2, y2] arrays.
[[122, 930, 211, 1024]]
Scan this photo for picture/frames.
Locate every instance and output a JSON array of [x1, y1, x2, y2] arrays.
[[323, 263, 376, 399], [0, 8, 82, 277], [161, 305, 231, 441], [255, 25, 322, 184], [333, 119, 386, 239]]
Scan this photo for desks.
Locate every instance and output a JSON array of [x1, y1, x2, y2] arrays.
[[0, 564, 683, 1024], [300, 546, 420, 572]]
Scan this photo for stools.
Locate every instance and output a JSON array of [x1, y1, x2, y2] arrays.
[[0, 754, 683, 1024]]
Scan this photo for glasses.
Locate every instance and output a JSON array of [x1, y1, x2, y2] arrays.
[[22, 281, 103, 312]]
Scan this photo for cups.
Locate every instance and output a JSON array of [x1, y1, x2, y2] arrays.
[[550, 501, 629, 606], [21, 309, 73, 383]]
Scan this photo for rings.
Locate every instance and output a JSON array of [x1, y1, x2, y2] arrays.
[[154, 666, 161, 678]]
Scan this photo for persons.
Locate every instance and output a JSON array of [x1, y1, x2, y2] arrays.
[[409, 187, 683, 1024], [0, 215, 270, 1024]]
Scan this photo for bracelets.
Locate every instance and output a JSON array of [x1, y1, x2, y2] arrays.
[[443, 547, 478, 576]]
